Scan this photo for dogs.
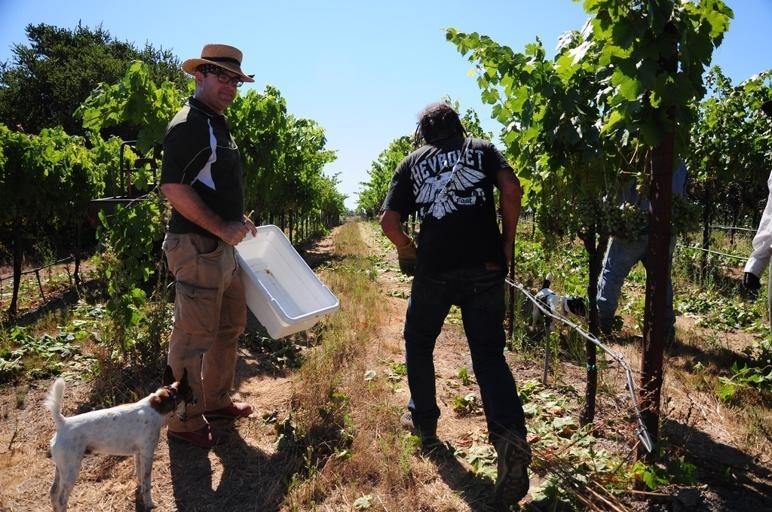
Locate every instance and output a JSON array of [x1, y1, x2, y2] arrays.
[[532, 272, 588, 332], [43, 365, 198, 512]]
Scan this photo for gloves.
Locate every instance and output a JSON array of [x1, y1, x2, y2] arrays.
[[742, 272, 761, 297]]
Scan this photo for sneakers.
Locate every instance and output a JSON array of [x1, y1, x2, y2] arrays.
[[495, 439, 529, 504], [411, 411, 437, 437]]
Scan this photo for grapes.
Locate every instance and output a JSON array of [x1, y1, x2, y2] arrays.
[[535, 188, 650, 240]]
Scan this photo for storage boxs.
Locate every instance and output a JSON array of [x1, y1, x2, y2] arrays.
[[231, 223, 342, 340]]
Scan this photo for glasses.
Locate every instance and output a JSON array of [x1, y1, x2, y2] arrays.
[[209, 70, 243, 87]]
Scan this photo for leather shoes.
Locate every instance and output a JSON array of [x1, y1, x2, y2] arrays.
[[167, 427, 230, 447], [204, 401, 252, 419]]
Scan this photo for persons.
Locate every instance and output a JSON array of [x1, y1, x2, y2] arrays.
[[597, 158, 688, 358], [379, 106, 532, 508], [742, 169, 772, 290], [159, 44, 257, 448]]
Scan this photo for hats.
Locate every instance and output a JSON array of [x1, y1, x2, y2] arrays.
[[182, 45, 254, 82]]
